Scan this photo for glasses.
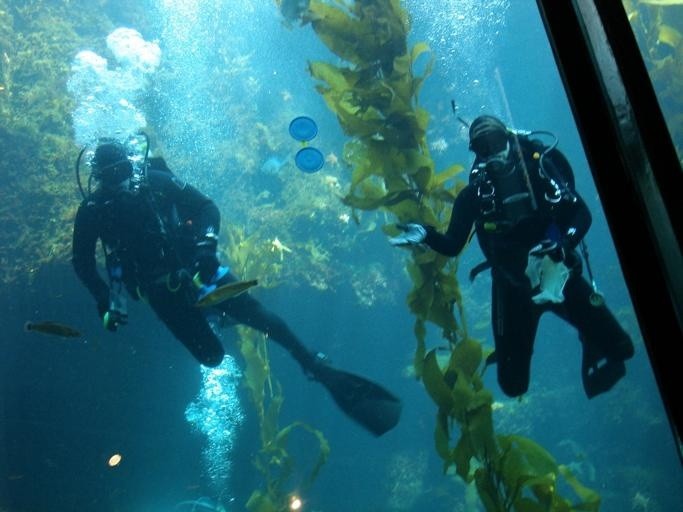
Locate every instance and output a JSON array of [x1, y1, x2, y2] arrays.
[[470, 131, 506, 157], [100, 159, 134, 184]]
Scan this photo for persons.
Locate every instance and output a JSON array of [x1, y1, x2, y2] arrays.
[[71, 142, 403, 438], [388, 113, 636, 403]]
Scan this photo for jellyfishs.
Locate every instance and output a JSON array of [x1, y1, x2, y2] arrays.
[[288, 494, 303, 512], [261, 156, 291, 176], [107, 453, 122, 468]]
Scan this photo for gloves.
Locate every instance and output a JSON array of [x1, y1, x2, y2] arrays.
[[187, 242, 220, 287], [97, 292, 128, 334], [386, 223, 427, 246], [528, 239, 567, 263]]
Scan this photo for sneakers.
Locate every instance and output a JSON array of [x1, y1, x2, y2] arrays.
[[313, 363, 343, 390]]
